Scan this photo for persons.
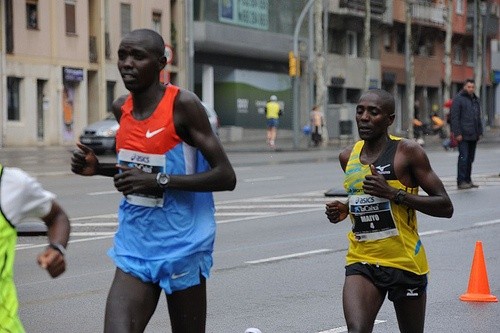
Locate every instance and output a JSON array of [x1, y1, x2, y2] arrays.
[[264, 95, 282, 146], [0, 166, 70, 333], [309, 104, 325, 145], [413, 100, 444, 135], [325, 89, 454, 333], [70, 29, 236, 333], [450, 79, 483, 189]]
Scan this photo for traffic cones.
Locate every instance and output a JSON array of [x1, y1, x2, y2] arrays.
[[458, 240, 499, 302]]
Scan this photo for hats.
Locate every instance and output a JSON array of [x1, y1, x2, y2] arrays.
[[270, 95, 277, 100]]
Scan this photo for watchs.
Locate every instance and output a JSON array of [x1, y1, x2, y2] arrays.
[[394, 190, 406, 204], [157, 173, 170, 191]]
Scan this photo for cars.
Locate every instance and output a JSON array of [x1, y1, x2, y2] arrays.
[[79, 101, 220, 155]]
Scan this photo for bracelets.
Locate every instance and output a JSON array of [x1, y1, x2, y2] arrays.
[[47, 243, 66, 255]]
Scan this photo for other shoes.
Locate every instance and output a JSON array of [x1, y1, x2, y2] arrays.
[[470, 182, 478, 188], [458, 183, 471, 189]]
